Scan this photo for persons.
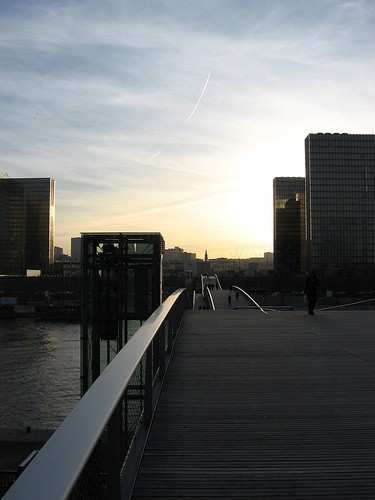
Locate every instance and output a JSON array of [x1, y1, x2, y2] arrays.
[[198, 305, 201, 309], [235, 292, 239, 301], [206, 305, 210, 309], [227, 295, 232, 306], [303, 271, 319, 315], [229, 284, 232, 292], [211, 283, 214, 291], [208, 284, 210, 290], [202, 305, 206, 309], [216, 282, 218, 290]]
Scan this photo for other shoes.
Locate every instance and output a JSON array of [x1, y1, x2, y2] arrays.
[[308, 311, 314, 315]]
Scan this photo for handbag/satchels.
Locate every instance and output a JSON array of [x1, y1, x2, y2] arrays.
[[303, 294, 306, 303]]
